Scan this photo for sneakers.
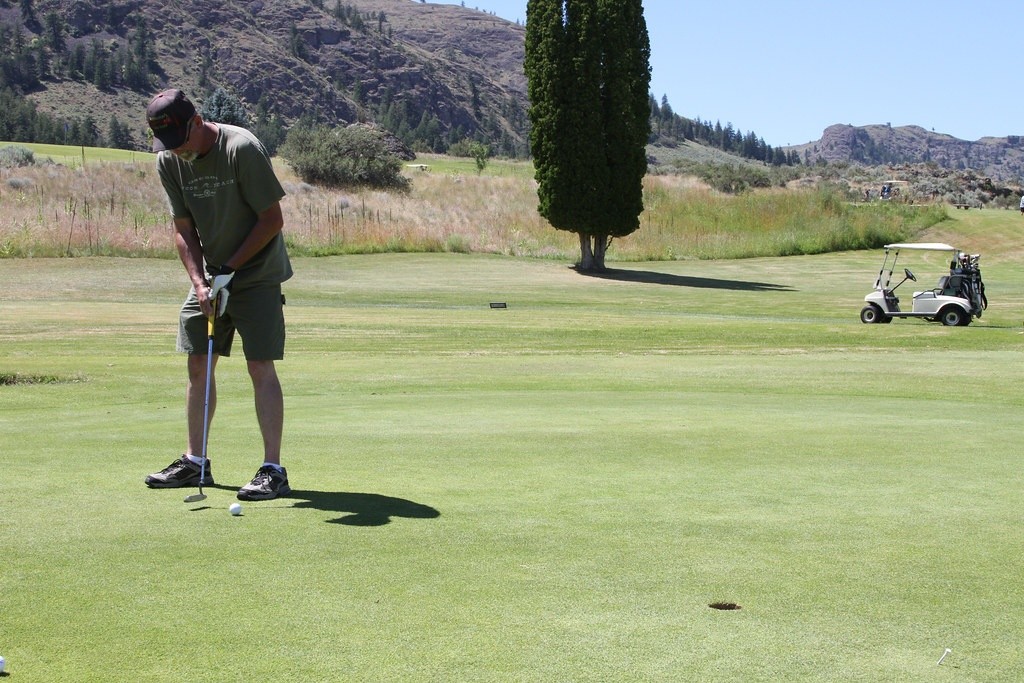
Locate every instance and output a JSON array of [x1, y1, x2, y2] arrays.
[[236, 465, 291, 500], [144, 453, 214, 488]]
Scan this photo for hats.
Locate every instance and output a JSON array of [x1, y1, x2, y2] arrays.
[[146, 87, 198, 153]]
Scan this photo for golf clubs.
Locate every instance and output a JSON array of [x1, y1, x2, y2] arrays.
[[184, 272, 220, 503]]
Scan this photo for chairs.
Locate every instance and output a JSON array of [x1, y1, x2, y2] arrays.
[[913, 275, 949, 298]]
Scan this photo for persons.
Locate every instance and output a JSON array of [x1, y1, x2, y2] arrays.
[[145, 90, 294, 501], [1020, 193, 1024, 215], [881, 183, 900, 196]]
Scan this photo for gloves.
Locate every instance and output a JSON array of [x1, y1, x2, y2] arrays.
[[207, 264, 236, 319]]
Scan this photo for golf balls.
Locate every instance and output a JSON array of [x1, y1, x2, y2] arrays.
[[229, 502, 244, 517]]
